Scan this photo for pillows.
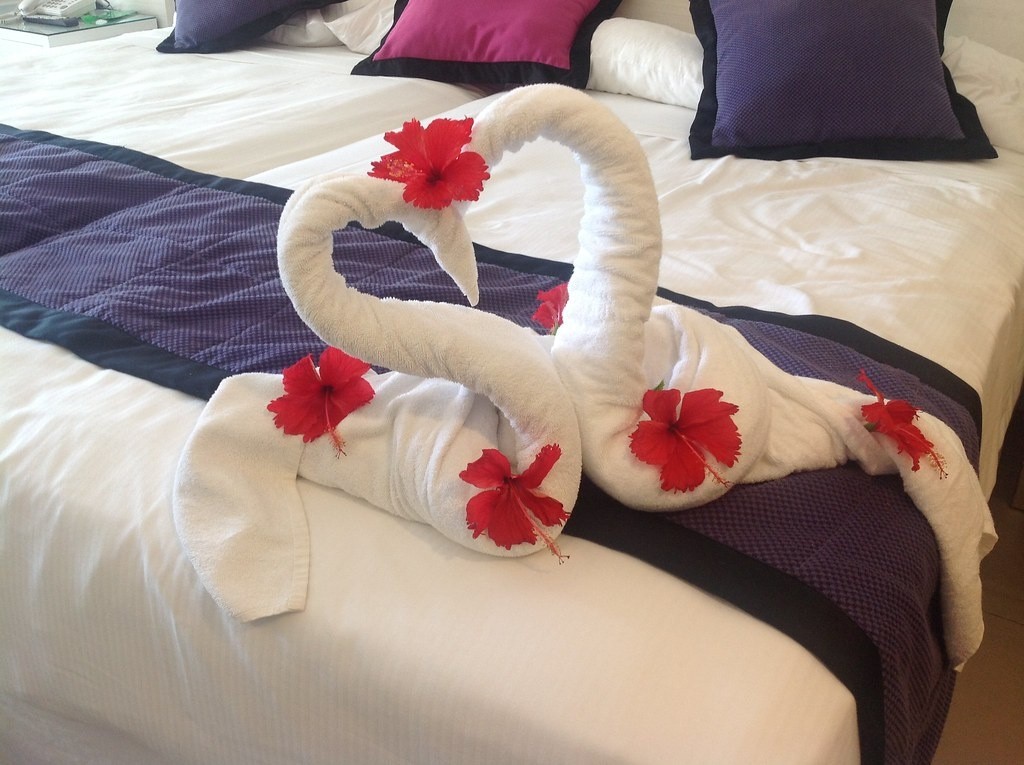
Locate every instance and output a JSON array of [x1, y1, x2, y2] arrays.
[[156, 0, 349, 54], [266, 8, 344, 47], [689, 0, 1000, 163], [322, 0, 397, 55], [585, 17, 704, 111], [350, 0, 624, 89]]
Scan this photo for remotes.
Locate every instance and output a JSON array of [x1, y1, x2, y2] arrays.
[[22, 15, 80, 27]]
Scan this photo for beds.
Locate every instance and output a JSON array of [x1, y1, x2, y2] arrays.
[[0, 0, 1024, 765]]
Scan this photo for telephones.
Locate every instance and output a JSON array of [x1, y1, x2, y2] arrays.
[[18, 0, 97, 19]]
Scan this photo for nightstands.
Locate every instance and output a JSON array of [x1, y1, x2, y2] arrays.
[[0, 9, 158, 48]]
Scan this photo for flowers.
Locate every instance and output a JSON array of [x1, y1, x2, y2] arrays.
[[255, 111, 946, 577]]
